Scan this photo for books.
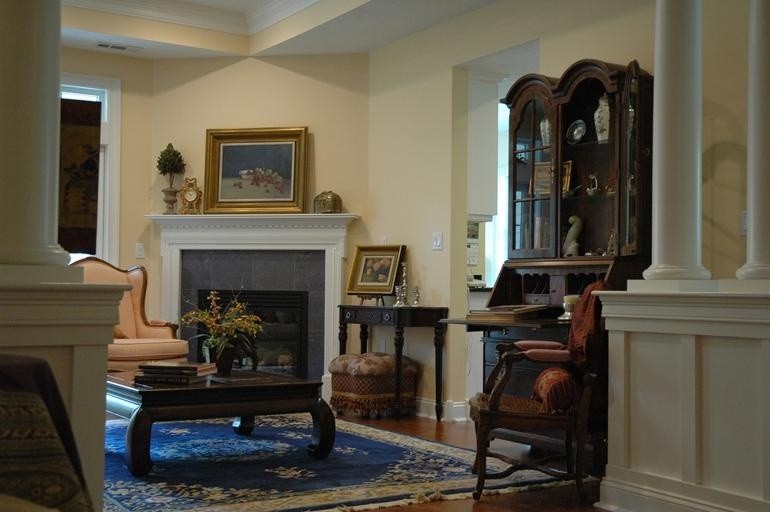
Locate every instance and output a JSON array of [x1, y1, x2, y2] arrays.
[[138, 358, 216, 370], [210, 371, 273, 384], [143, 368, 218, 376], [468, 301, 567, 323], [134, 374, 209, 385]]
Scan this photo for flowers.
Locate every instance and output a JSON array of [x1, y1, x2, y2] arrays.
[[178, 283, 270, 370]]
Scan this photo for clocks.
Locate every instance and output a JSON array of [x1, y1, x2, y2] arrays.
[[177, 177, 203, 215]]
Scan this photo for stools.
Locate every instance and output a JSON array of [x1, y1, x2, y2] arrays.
[[328, 352, 420, 419]]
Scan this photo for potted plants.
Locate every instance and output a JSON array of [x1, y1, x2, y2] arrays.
[[156, 142, 186, 215]]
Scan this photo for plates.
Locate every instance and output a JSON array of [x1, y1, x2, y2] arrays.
[[564, 120, 588, 145]]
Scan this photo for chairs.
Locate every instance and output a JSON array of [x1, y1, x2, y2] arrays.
[[466, 280, 611, 502], [68, 256, 190, 372]]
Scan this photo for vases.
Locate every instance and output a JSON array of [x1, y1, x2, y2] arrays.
[[214, 344, 234, 376]]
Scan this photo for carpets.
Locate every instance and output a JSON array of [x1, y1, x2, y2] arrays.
[[104, 410, 601, 511]]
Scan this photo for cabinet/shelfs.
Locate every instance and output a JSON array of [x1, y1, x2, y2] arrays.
[[439, 59, 653, 474]]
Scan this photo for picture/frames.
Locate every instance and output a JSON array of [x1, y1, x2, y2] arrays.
[[343, 244, 406, 296], [203, 127, 308, 214]]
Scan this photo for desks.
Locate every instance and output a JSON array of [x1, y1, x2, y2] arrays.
[[336, 305, 449, 424]]
